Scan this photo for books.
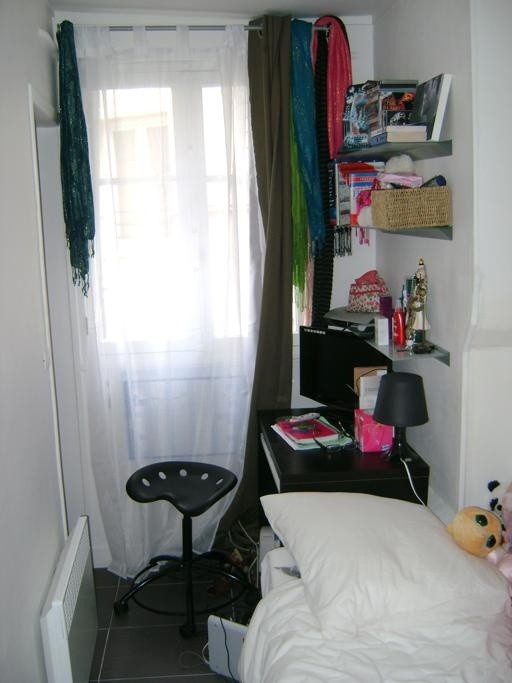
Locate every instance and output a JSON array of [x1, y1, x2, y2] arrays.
[[328, 157, 385, 225], [270, 415, 353, 451], [370, 132, 428, 146], [371, 124, 428, 138], [410, 72, 452, 141]]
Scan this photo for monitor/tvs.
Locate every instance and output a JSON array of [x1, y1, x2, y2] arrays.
[[300, 325, 392, 423]]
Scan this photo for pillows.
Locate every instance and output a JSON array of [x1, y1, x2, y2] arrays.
[[258, 486, 511, 638]]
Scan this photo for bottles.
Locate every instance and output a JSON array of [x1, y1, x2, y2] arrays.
[[390, 258, 430, 352]]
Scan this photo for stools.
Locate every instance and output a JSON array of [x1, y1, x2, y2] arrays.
[[110, 458, 263, 638]]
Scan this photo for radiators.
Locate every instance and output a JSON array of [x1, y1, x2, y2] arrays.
[[33, 514, 103, 682]]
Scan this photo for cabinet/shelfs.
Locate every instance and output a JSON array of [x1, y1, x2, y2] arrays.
[[253, 408, 430, 507], [332, 137, 456, 367]]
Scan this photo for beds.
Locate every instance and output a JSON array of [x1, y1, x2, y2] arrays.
[[230, 527, 511, 683]]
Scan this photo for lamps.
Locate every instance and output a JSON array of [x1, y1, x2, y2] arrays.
[[369, 369, 429, 464]]
